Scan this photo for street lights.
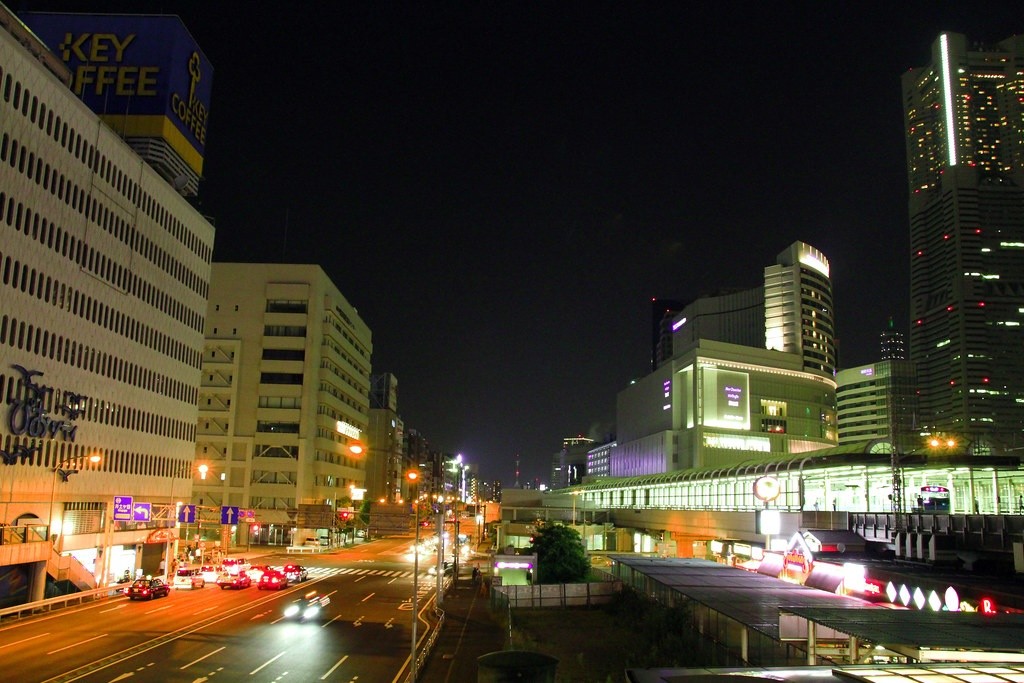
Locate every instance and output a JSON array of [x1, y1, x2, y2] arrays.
[[164, 465, 208, 583], [406, 469, 422, 683], [47, 455, 101, 539]]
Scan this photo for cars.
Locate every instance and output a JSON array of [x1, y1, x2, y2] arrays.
[[174, 567, 205, 589], [285, 564, 309, 584], [204, 558, 288, 591], [124, 576, 169, 601]]
